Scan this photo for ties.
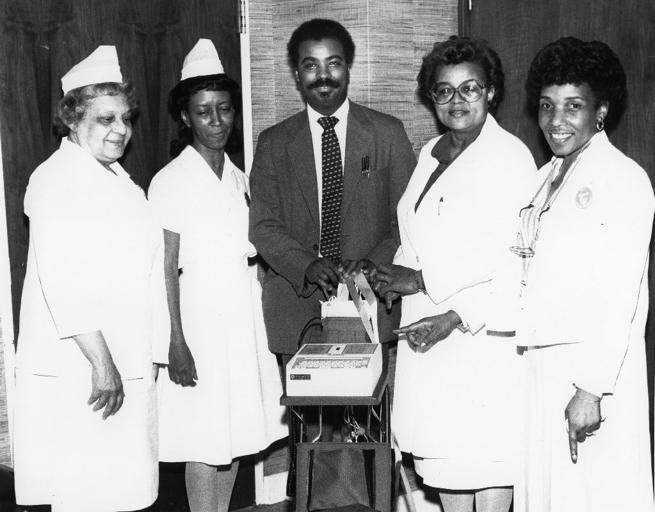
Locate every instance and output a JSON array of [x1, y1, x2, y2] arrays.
[[318, 117, 344, 265]]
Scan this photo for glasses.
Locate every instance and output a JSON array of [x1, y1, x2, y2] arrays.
[[429, 80, 487, 105], [510, 205, 551, 259]]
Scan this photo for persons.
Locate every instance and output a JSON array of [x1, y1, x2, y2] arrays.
[[146, 75, 289, 512], [249, 20, 418, 386], [391, 36, 655, 512], [381, 36, 538, 511], [11, 82, 168, 511]]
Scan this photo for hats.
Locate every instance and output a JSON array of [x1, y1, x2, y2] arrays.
[[181, 39, 225, 80], [61, 44, 123, 96]]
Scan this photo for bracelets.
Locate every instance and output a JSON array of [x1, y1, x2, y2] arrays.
[[574, 393, 600, 406]]
